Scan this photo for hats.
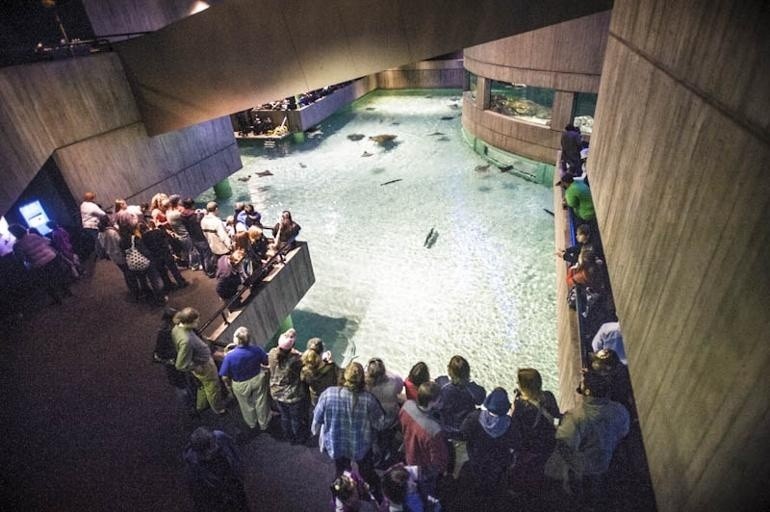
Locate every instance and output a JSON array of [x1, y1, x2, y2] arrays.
[[278, 328, 296, 350], [587, 348, 619, 366], [484, 388, 509, 415], [307, 337, 324, 354], [342, 362, 365, 387], [576, 373, 609, 398], [182, 426, 225, 464], [229, 249, 247, 269]]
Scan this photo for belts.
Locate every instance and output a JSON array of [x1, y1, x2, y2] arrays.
[[233, 372, 260, 382]]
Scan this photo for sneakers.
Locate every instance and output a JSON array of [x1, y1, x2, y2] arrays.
[[198, 263, 203, 271], [179, 281, 189, 288], [167, 282, 177, 287], [192, 266, 198, 271], [159, 295, 168, 307]]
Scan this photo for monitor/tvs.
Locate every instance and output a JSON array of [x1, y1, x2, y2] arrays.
[[0, 216, 16, 259], [19, 200, 54, 236]]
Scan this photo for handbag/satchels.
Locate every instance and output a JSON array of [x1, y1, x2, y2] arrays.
[[273, 221, 281, 250], [127, 235, 150, 272], [527, 397, 557, 447]]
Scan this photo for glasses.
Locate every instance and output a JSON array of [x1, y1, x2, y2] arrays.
[[517, 381, 520, 384]]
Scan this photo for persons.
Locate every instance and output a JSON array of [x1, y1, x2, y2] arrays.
[[561, 124, 582, 173], [234, 202, 247, 234], [169, 308, 228, 416], [165, 194, 195, 269], [512, 367, 561, 468], [311, 362, 386, 480], [28, 228, 43, 238], [328, 470, 378, 511], [153, 307, 198, 413], [561, 174, 595, 226], [399, 382, 449, 486], [118, 222, 169, 308], [309, 339, 325, 354], [566, 247, 607, 313], [247, 224, 270, 272], [438, 388, 514, 490], [580, 348, 631, 407], [271, 211, 301, 252], [363, 358, 404, 464], [439, 355, 486, 427], [140, 202, 152, 222], [225, 216, 235, 237], [231, 231, 266, 283], [180, 197, 216, 278], [200, 201, 234, 257], [137, 221, 190, 290], [80, 191, 111, 261], [556, 222, 596, 264], [543, 370, 631, 502], [405, 362, 429, 400], [219, 326, 273, 431], [299, 350, 336, 407], [593, 322, 628, 366], [185, 428, 249, 512], [249, 86, 338, 112], [381, 462, 443, 512], [46, 220, 85, 278], [97, 215, 140, 296], [112, 198, 138, 226], [150, 193, 168, 229], [215, 248, 248, 313], [7, 222, 74, 305], [236, 205, 264, 230], [267, 327, 307, 440]]
